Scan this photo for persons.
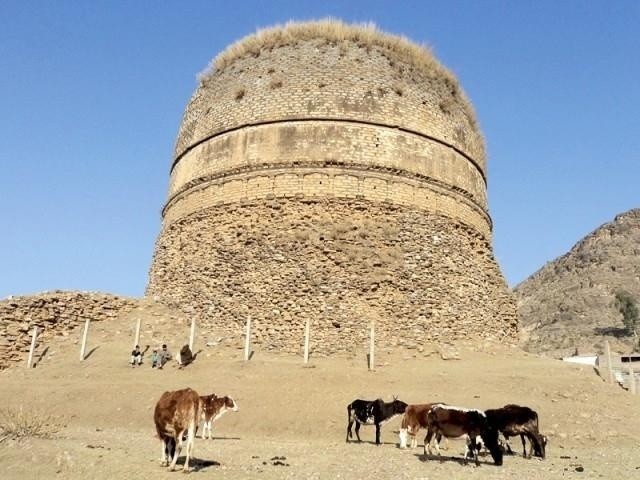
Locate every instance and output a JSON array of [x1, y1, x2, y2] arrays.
[[129, 345, 144, 365], [158, 344, 172, 369], [151, 351, 161, 367]]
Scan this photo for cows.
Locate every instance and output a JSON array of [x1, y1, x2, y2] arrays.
[[199, 393, 217, 402], [346, 394, 408, 444], [393, 403, 449, 451], [424, 405, 547, 466], [196, 396, 239, 440], [155, 388, 204, 473]]
